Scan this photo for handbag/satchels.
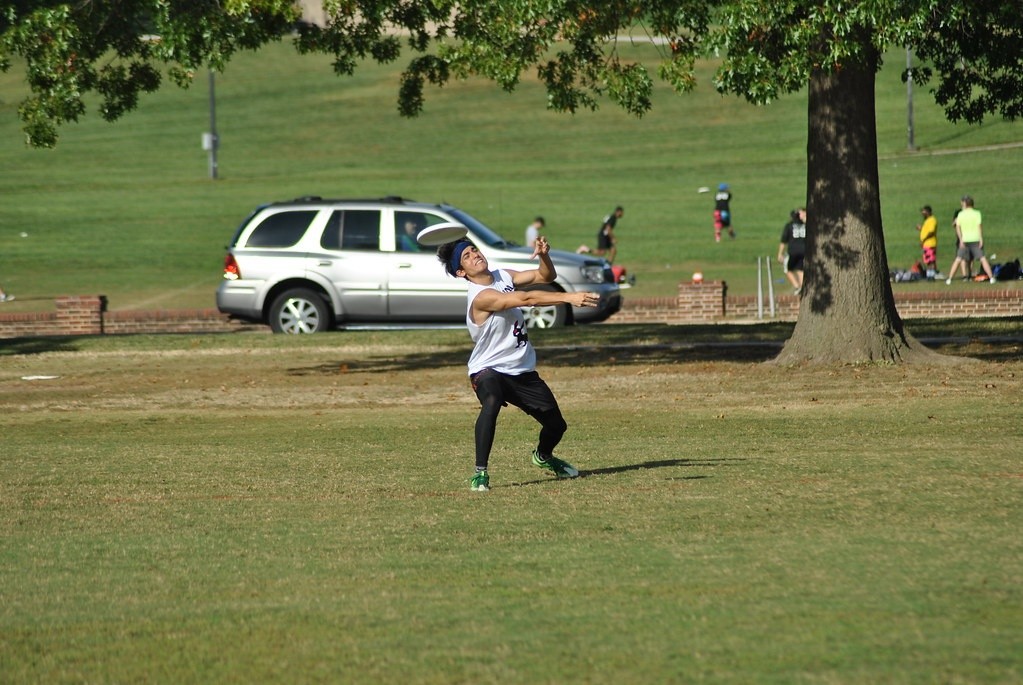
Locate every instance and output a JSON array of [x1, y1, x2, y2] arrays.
[[890, 261, 924, 281], [981, 260, 1022, 281]]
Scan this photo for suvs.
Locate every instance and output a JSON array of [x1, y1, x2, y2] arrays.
[[215, 195, 624, 333]]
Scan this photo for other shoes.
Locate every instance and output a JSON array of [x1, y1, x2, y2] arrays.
[[947, 279, 951, 285], [989, 279, 996, 284], [793, 288, 801, 294]]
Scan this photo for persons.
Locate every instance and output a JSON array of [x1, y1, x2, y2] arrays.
[[916, 205, 938, 283], [799, 207, 806, 223], [525, 217, 545, 247], [437, 233, 602, 491], [953, 196, 975, 281], [575, 205, 623, 264], [777, 210, 807, 296], [712, 183, 735, 242], [945, 198, 996, 285]]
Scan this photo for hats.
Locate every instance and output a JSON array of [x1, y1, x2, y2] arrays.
[[719, 184, 727, 190], [920, 206, 932, 213]]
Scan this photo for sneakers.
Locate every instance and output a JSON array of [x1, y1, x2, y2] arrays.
[[531, 450, 579, 479], [468, 470, 491, 493]]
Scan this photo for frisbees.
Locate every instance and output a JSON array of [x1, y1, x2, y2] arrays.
[[697, 187, 709, 194], [417, 222, 468, 246]]
